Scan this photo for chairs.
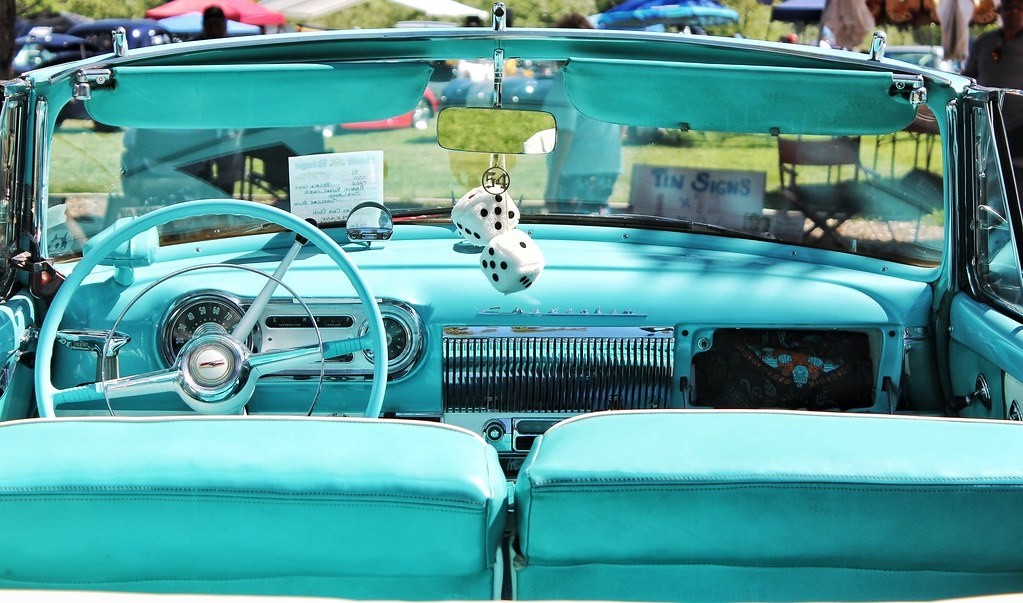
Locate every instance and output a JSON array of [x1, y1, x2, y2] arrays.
[[510, 412, 1023, 601], [773, 135, 937, 254], [0, 415, 506, 598]]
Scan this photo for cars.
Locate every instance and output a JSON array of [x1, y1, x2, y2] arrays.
[[9, 17, 173, 132], [869, 43, 968, 134], [437, 66, 554, 110]]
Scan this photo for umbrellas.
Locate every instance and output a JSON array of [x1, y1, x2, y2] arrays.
[[598, 0, 738, 32]]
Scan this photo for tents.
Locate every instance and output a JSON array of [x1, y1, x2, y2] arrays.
[[258, 0, 489, 33], [765, 0, 826, 47], [146, 0, 285, 35]]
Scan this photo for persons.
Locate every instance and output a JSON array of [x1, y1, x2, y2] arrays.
[[541, 13, 624, 214], [121, 6, 323, 205], [959, 0, 1023, 270], [456, 16, 495, 82]]
